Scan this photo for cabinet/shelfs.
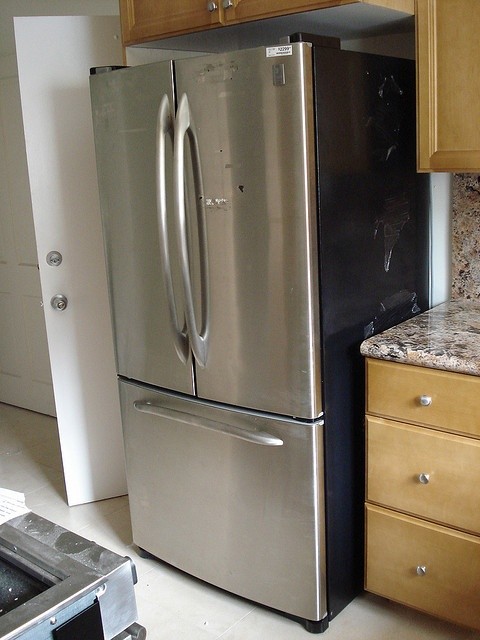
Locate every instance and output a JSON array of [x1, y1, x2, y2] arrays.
[[119, 0, 412, 61], [364, 354, 480, 632], [412, 0, 480, 175]]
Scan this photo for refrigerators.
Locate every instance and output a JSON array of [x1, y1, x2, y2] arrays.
[[87, 25, 432, 635]]
